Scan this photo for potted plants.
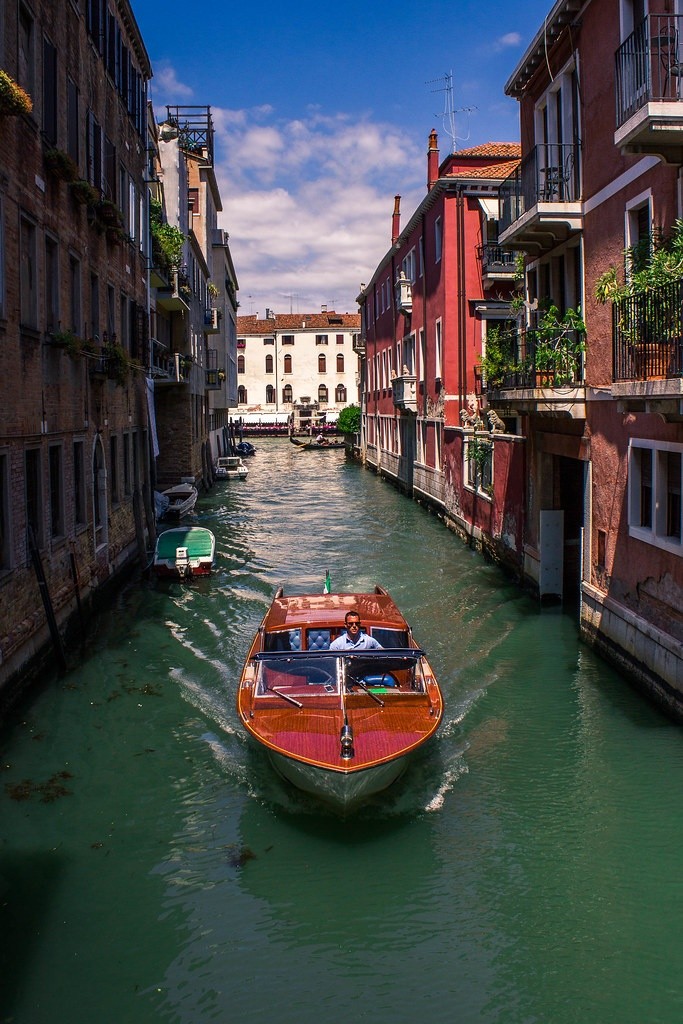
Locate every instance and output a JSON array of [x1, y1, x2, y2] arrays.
[[532, 304, 588, 390], [70, 175, 99, 204], [595, 222, 683, 381], [96, 197, 122, 221], [107, 226, 127, 247], [44, 147, 80, 184]]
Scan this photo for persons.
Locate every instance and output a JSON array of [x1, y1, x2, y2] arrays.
[[334, 438, 338, 443], [305, 423, 310, 431], [327, 611, 384, 686], [316, 433, 326, 444]]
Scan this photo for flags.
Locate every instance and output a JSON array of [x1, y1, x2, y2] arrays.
[[323, 574, 330, 594]]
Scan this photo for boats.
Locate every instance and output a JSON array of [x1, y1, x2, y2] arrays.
[[215, 454, 248, 481], [290, 432, 346, 449], [236, 578, 445, 819], [158, 482, 198, 522], [153, 527, 216, 581], [235, 440, 256, 455]]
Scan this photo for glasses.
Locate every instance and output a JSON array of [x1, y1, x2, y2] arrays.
[[346, 622, 361, 625]]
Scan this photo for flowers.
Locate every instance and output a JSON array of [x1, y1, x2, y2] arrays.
[[0, 66, 33, 115]]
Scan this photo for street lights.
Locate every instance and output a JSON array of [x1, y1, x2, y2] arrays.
[[273, 329, 278, 411]]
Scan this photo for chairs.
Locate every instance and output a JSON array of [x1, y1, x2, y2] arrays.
[[287, 629, 330, 678]]
